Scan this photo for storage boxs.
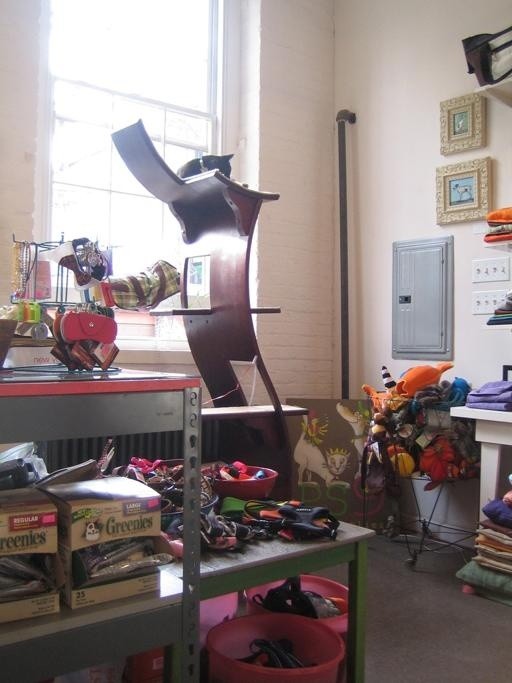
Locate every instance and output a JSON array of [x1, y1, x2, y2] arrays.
[[2, 487, 57, 624], [39, 475, 166, 611]]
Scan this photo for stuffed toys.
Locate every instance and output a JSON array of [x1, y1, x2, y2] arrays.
[[355, 361, 482, 492]]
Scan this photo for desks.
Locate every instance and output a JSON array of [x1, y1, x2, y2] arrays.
[[163, 506, 373, 679]]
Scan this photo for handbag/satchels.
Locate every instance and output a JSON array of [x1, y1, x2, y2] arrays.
[[110, 262, 180, 311]]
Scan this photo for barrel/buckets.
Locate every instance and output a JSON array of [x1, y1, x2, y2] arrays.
[[206, 612, 346, 683], [243, 576, 349, 683]]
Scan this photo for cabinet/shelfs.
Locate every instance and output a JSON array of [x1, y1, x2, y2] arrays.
[[2, 364, 202, 682], [450, 241, 512, 557], [367, 393, 481, 570], [127, 165, 310, 502]]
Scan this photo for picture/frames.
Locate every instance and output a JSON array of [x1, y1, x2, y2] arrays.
[[435, 156, 494, 225], [439, 92, 488, 156]]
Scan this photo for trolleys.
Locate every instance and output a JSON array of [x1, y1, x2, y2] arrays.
[[360, 459, 480, 570]]
